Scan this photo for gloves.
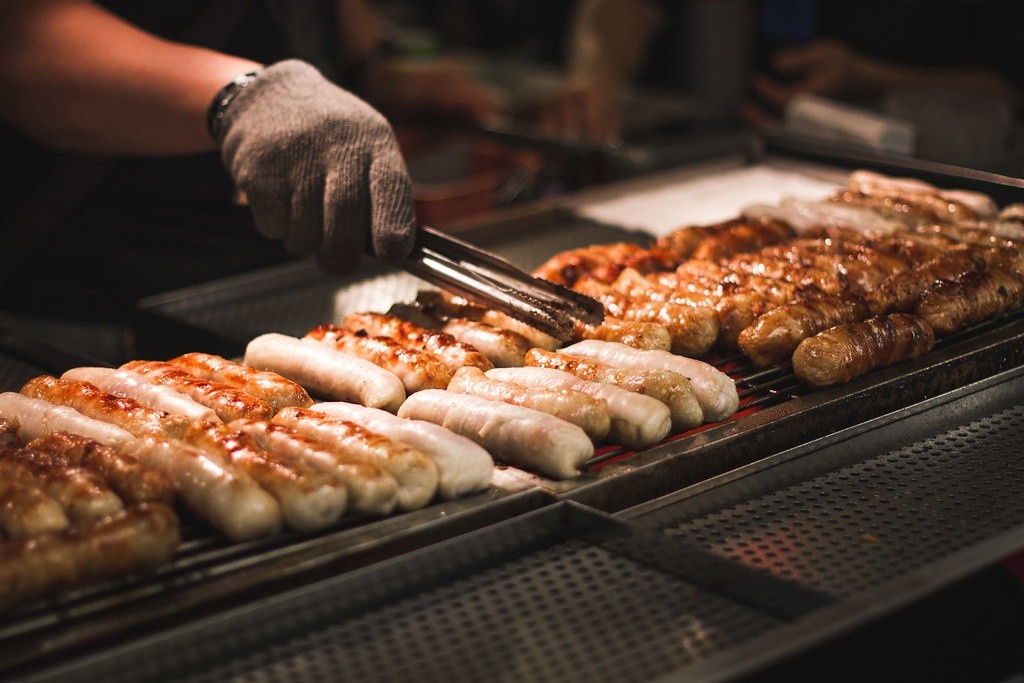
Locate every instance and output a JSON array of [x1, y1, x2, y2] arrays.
[[215, 56, 418, 263]]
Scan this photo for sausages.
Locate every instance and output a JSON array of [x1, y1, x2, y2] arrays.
[[0, 168, 1024, 606]]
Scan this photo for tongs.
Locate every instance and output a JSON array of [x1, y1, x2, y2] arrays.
[[359, 210, 608, 340]]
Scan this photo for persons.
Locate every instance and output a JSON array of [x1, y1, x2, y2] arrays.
[[339, 0, 696, 147], [2, 0, 416, 278]]
